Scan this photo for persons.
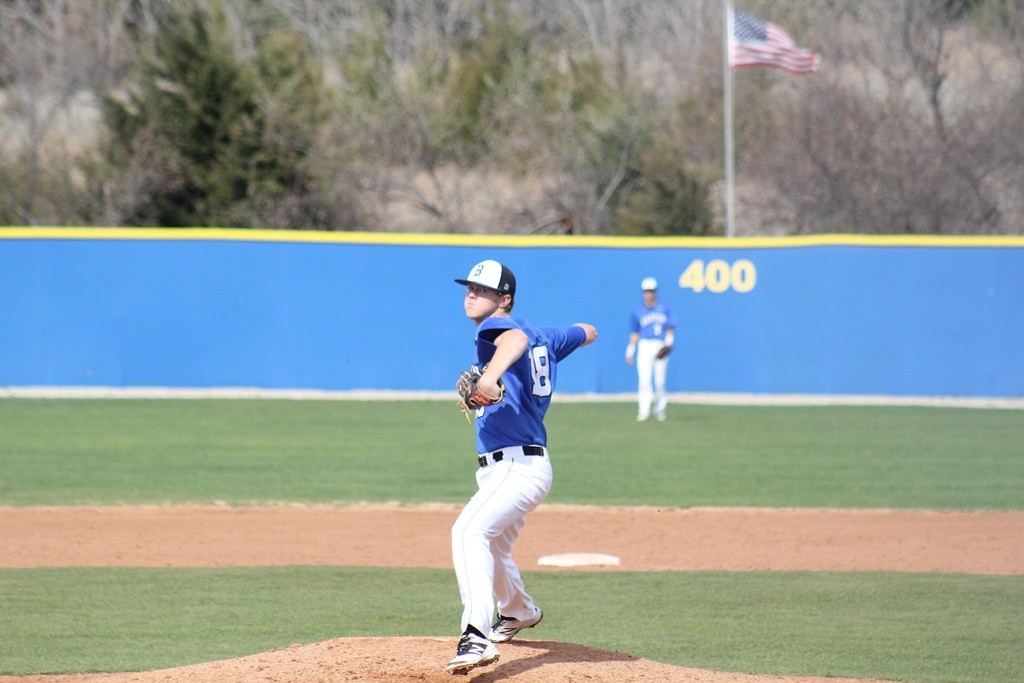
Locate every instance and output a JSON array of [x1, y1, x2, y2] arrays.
[[626, 277, 676, 422], [447, 259, 596, 672]]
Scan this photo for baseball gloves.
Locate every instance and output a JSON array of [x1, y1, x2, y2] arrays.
[[657, 346, 671, 359], [456, 363, 507, 410]]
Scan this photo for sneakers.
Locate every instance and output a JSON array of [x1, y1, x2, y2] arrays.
[[446, 633, 500, 676], [652, 408, 666, 422], [488, 607, 543, 644], [636, 412, 650, 422]]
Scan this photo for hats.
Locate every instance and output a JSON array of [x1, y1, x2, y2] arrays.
[[641, 277, 657, 290], [454, 260, 516, 297]]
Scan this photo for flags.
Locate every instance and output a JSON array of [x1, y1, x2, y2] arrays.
[[733, 11, 819, 74]]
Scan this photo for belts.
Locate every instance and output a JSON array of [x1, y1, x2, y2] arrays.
[[478, 446, 544, 467]]
